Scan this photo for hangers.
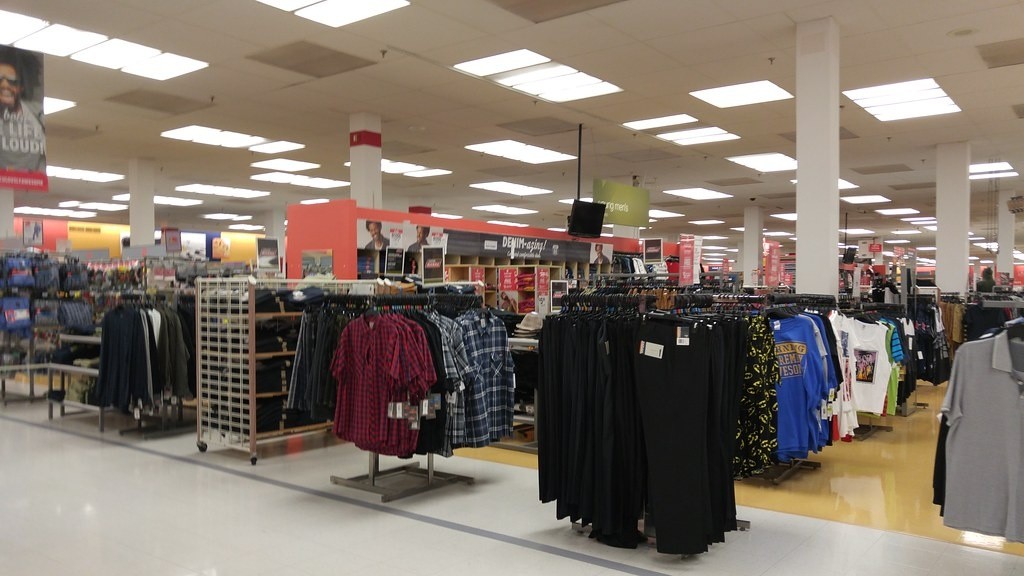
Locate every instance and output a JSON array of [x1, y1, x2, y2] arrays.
[[117, 281, 1024, 342]]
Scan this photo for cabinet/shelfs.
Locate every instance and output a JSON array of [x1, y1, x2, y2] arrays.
[[46, 333, 119, 434], [283, 197, 679, 316], [191, 277, 377, 463]]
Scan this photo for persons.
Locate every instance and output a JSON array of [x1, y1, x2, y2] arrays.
[[977, 267, 995, 292], [32, 222, 40, 240], [408, 225, 430, 253], [501, 292, 517, 314], [0, 45, 46, 173], [365, 221, 390, 250], [594, 244, 609, 263]]
